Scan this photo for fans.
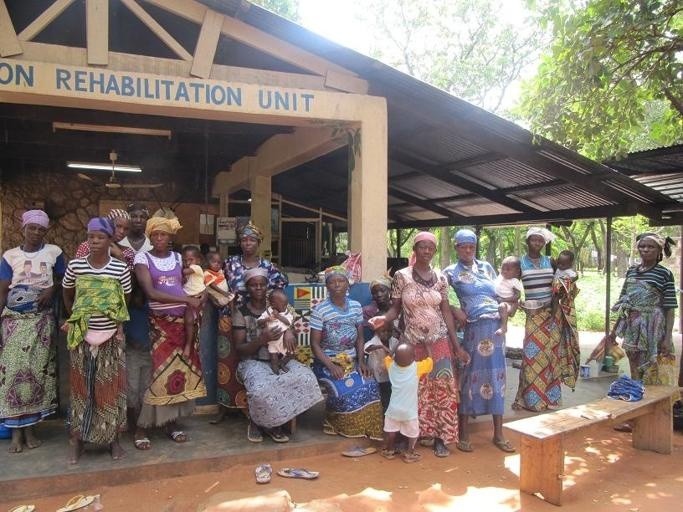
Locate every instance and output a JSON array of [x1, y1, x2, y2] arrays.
[[75, 151, 166, 190]]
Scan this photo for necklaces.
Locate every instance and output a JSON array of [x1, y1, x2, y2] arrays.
[[23, 243, 42, 259]]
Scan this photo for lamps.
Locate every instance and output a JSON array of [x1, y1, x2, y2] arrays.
[[65, 159, 145, 175]]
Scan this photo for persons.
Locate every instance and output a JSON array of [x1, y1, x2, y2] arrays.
[[368, 231, 471, 458], [550, 250, 578, 322], [0, 209, 66, 453], [441, 229, 518, 452], [19, 260, 36, 278], [511, 226, 580, 412], [494, 256, 521, 336], [380, 335, 434, 464], [611, 232, 679, 431], [38, 262, 48, 278]]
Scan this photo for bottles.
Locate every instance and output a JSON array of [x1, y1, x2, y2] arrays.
[[604, 356, 614, 366], [579, 365, 591, 378]]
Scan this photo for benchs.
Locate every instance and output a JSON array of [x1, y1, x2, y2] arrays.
[[500, 385, 675, 506]]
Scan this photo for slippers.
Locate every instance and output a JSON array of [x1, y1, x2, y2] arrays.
[[455, 438, 476, 453], [164, 427, 191, 444], [276, 466, 320, 480], [246, 423, 265, 444], [265, 428, 289, 443], [321, 418, 452, 465], [254, 463, 272, 485], [492, 438, 518, 453], [131, 431, 155, 452]]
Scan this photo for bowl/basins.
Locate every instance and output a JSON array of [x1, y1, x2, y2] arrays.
[[521, 300, 544, 315]]
